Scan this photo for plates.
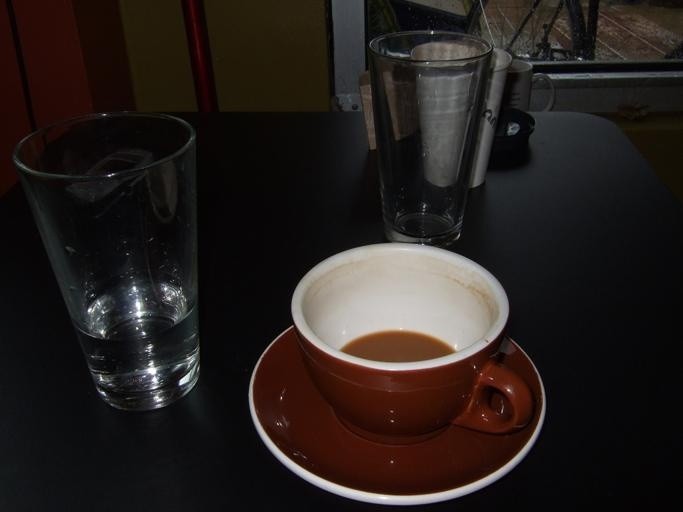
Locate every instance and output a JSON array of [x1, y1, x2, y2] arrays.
[[251, 324, 546, 507]]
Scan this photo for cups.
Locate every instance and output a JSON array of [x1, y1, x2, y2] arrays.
[[291, 244, 535, 445], [494, 57, 555, 166], [409, 40, 512, 189], [13, 110, 202, 411], [369, 30, 494, 244]]
